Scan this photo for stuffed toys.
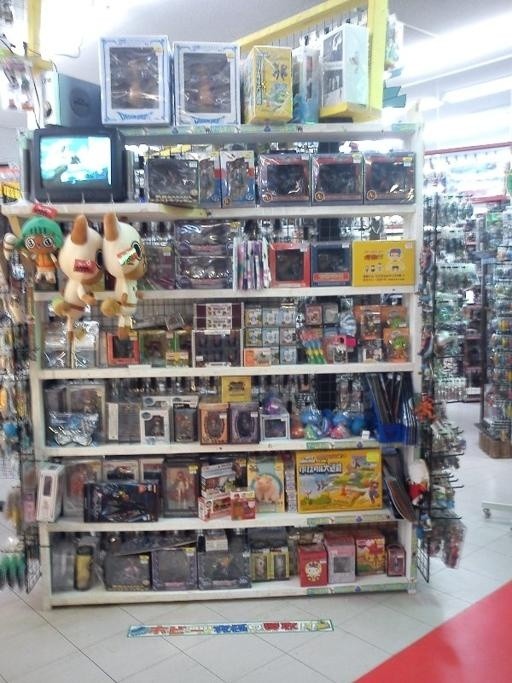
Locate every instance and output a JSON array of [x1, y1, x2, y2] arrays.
[[20, 216, 62, 287], [98, 212, 149, 344], [58, 215, 106, 328]]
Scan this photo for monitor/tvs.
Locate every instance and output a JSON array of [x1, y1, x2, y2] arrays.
[[33, 128, 127, 201]]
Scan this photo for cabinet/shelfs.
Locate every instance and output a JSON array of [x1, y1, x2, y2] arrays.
[[20, 122, 421, 610]]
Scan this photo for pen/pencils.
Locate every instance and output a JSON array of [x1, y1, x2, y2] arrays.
[[237, 241, 245, 290]]
